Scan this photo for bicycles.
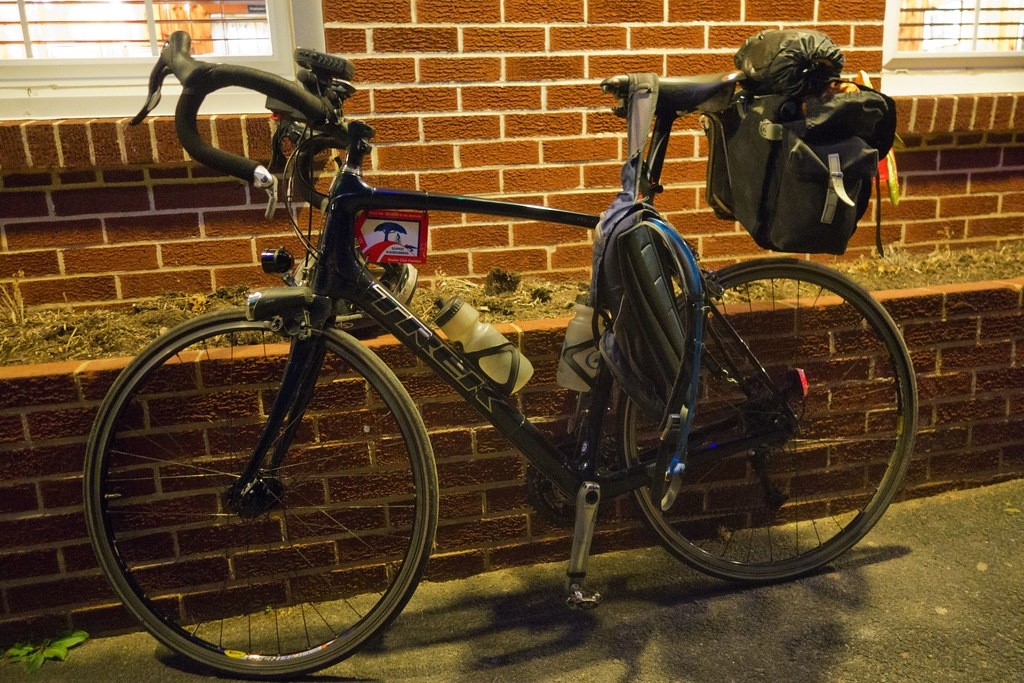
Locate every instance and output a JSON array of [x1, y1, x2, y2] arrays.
[[82, 31, 919, 681]]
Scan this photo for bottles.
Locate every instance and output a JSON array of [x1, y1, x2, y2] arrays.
[[431, 295, 539, 397], [556, 290, 607, 395]]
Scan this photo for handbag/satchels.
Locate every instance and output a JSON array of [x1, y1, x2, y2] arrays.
[[700, 83, 897, 257]]
